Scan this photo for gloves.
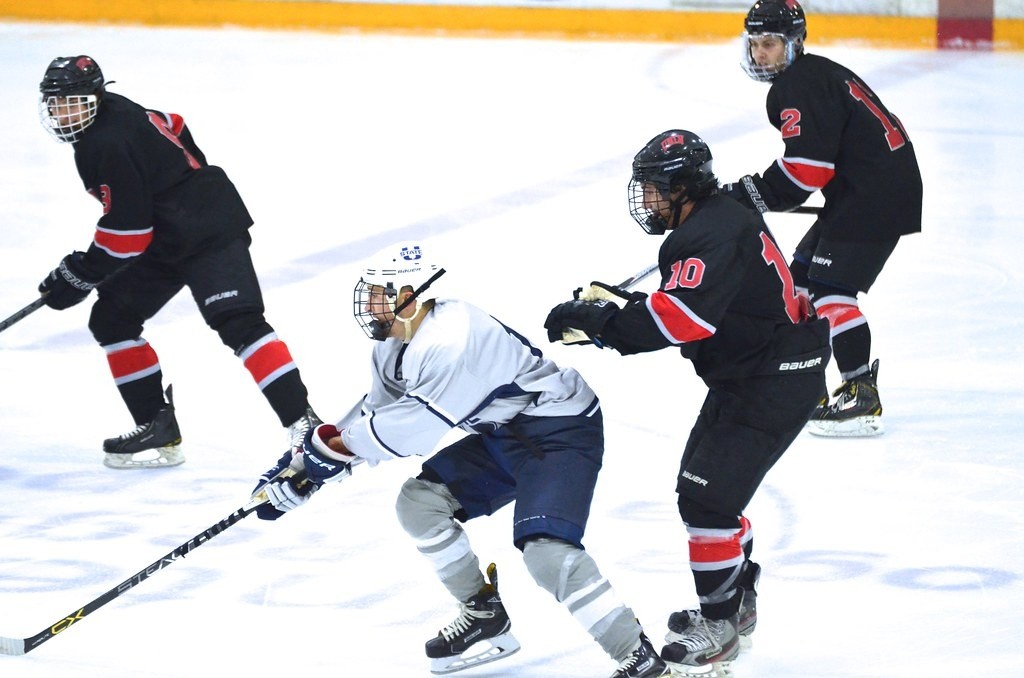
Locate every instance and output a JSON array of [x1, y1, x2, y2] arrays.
[[544, 297, 619, 350], [38, 250, 101, 312], [250, 446, 353, 522], [573, 280, 650, 311], [264, 424, 339, 511]]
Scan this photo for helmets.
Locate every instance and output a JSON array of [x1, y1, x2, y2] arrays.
[[740, 0, 807, 81], [40, 55, 104, 141], [628, 129, 714, 236], [352, 240, 446, 342]]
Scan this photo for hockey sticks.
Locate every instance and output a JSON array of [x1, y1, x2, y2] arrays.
[[790, 203, 824, 215], [615, 263, 659, 290], [0, 470, 310, 656], [0, 294, 54, 331]]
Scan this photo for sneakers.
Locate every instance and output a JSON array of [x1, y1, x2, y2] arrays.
[[102, 384, 186, 469], [426, 562, 522, 676], [610, 618, 672, 678], [659, 586, 745, 676], [664, 562, 762, 652], [287, 406, 325, 452], [806, 358, 884, 435]]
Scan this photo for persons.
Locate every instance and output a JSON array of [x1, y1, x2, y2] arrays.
[[37, 53, 344, 470], [541, 125, 832, 675], [252, 240, 675, 678], [716, 0, 924, 436]]
